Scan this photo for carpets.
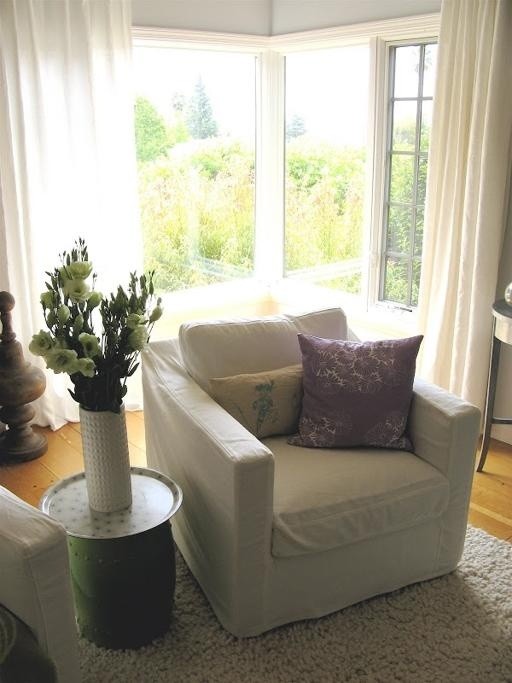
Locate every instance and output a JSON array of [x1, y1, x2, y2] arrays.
[[75, 523, 511, 683]]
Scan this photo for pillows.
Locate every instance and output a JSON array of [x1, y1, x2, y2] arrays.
[[178, 307, 348, 394], [288, 331, 423, 452], [208, 364, 303, 439]]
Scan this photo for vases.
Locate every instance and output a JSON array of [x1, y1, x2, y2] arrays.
[[78, 401, 132, 512]]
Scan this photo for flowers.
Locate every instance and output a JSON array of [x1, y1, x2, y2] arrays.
[[28, 237, 163, 413]]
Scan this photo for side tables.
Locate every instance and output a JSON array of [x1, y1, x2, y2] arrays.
[[39, 466, 183, 650], [476, 298, 512, 474]]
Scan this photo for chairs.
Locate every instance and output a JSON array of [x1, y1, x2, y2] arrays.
[[0, 484, 83, 683], [140, 327, 480, 639]]
